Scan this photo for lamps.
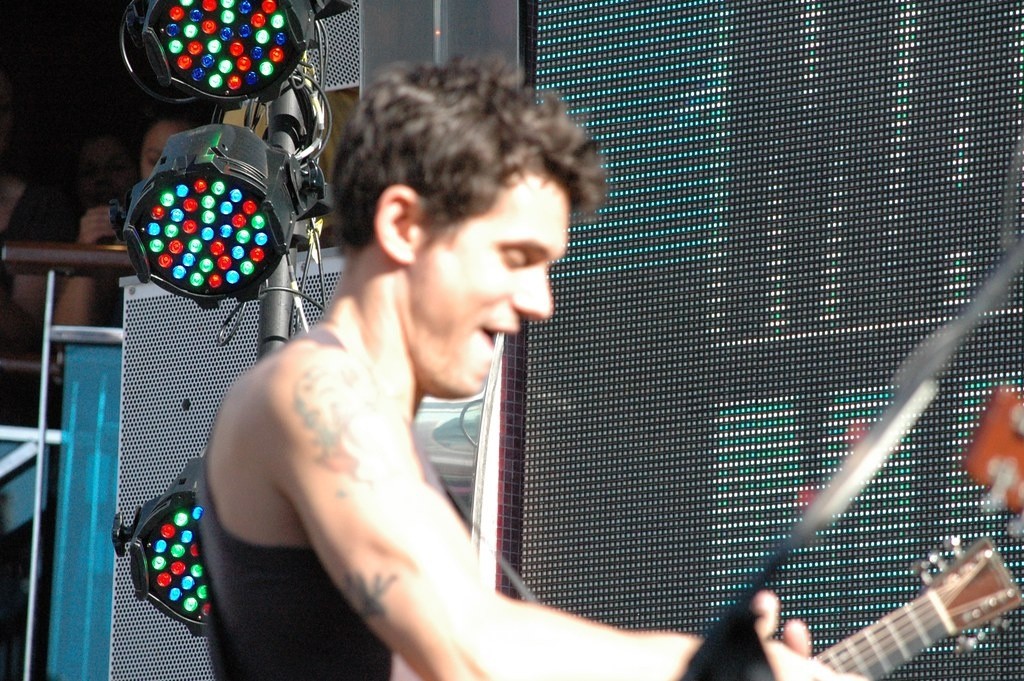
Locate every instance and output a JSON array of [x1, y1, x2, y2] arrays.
[[110, 124, 333, 311], [112, 454, 210, 638], [119, 0, 350, 109]]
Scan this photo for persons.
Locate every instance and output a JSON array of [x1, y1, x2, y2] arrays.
[[199, 52, 872, 681]]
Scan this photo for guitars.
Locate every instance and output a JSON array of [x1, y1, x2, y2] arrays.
[[815, 533, 1024, 680]]
